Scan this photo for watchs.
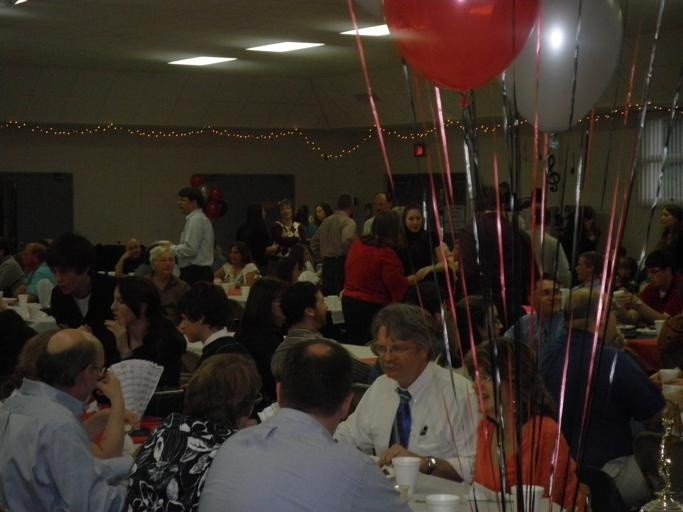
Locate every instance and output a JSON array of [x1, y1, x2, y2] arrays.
[[633, 298, 643, 310], [425, 455, 438, 475]]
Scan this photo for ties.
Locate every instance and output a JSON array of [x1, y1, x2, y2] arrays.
[[388, 388, 412, 449]]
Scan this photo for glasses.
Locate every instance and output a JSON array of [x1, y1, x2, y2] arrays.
[[480, 373, 494, 383], [370, 343, 418, 354], [535, 289, 560, 296], [93, 365, 107, 377]]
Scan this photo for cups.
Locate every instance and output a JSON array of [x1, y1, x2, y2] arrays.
[[510, 485, 545, 511], [428, 495, 459, 510], [391, 456, 418, 494]]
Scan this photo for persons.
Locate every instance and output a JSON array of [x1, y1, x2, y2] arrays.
[[360, 191, 393, 235], [82, 369, 139, 461], [270, 199, 308, 245], [394, 203, 455, 317], [235, 279, 284, 423], [614, 256, 640, 294], [270, 280, 329, 377], [0, 238, 26, 298], [499, 181, 518, 212], [309, 194, 358, 296], [44, 232, 119, 363], [341, 209, 416, 348], [1, 328, 139, 511], [178, 283, 251, 368], [168, 188, 215, 286], [521, 187, 543, 229], [234, 201, 270, 263], [613, 248, 683, 323], [119, 353, 263, 511], [575, 251, 606, 287], [308, 200, 334, 240], [147, 246, 188, 326], [458, 200, 531, 337], [296, 204, 317, 240], [536, 286, 666, 512], [524, 207, 572, 288], [650, 203, 683, 248], [331, 303, 481, 486], [212, 242, 262, 289], [436, 295, 503, 372], [102, 275, 186, 386], [503, 272, 563, 377], [466, 338, 588, 512], [114, 237, 147, 276], [265, 235, 305, 284], [13, 242, 58, 302]]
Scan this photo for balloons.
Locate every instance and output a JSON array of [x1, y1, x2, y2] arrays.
[[208, 184, 225, 201], [380, 1, 542, 108], [204, 201, 220, 221], [218, 201, 229, 217], [498, 0, 624, 137], [190, 174, 205, 189], [198, 184, 210, 209]]
